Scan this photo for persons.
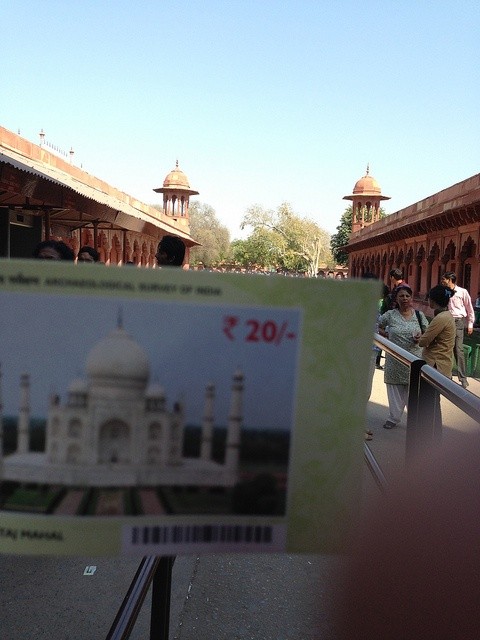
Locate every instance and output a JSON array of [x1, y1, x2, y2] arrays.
[[361, 269, 382, 442], [78, 245, 100, 263], [375, 269, 404, 369], [377, 283, 429, 429], [419, 285, 456, 430], [32, 239, 76, 262], [155, 235, 186, 267], [189, 265, 344, 281], [441, 271, 475, 388]]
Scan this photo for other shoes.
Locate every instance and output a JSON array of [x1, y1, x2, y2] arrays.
[[365, 434, 372, 440], [367, 430, 373, 435], [462, 382, 469, 388], [384, 421, 395, 429]]
